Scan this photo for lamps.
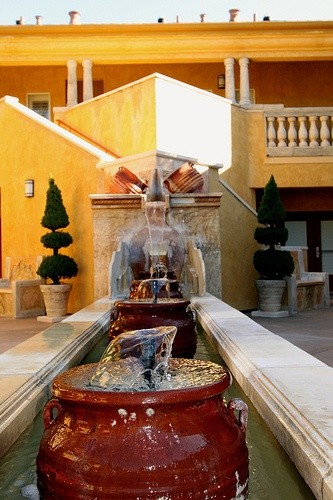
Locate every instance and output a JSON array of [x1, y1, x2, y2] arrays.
[[24, 179, 34, 198], [218, 75, 225, 88]]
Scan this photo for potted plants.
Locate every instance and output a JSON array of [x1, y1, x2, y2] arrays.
[[252, 174, 291, 318], [36, 173, 78, 323]]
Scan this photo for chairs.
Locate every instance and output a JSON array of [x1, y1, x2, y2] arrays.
[[0, 255, 44, 320], [280, 245, 330, 311]]
